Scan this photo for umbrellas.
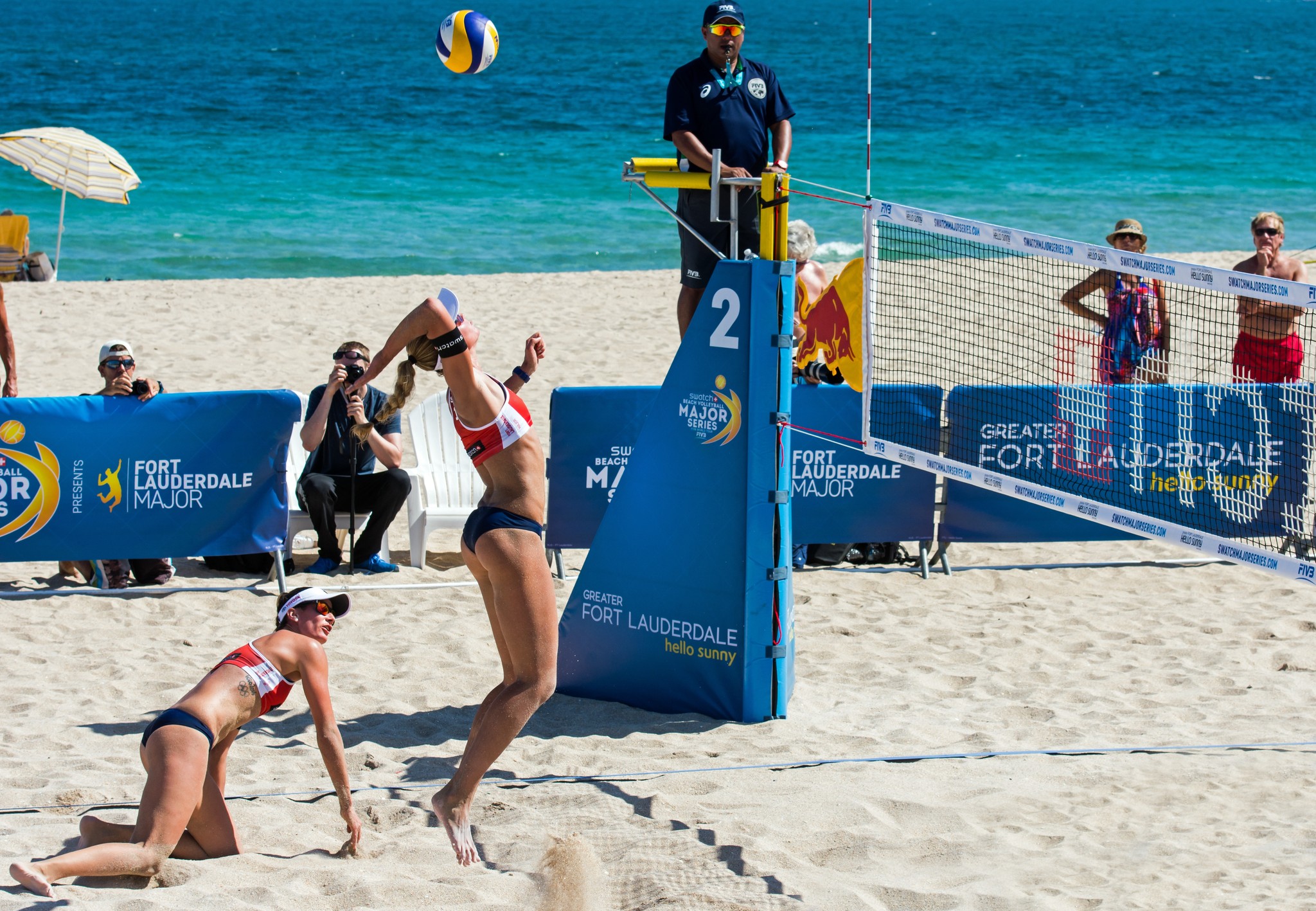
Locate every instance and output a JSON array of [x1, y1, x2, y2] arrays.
[[0, 126, 142, 281]]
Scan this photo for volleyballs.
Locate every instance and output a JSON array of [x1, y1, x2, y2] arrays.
[[435, 10, 499, 74]]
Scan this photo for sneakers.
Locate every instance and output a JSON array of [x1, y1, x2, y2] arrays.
[[302, 554, 339, 575], [349, 551, 399, 574]]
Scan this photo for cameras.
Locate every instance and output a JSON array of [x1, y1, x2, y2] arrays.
[[342, 364, 363, 385], [793, 356, 845, 386], [128, 380, 149, 395]]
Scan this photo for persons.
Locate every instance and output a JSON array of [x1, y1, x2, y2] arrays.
[[1232, 211, 1311, 385], [0, 210, 29, 262], [786, 219, 828, 385], [345, 289, 557, 867], [295, 342, 411, 574], [57, 339, 176, 590], [0, 283, 18, 398], [663, 0, 797, 345], [1059, 218, 1174, 388], [8, 586, 362, 898]]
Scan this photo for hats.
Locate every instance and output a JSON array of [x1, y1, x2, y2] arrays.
[[99, 340, 135, 361], [1105, 218, 1147, 246], [703, 0, 746, 26], [277, 586, 355, 623], [431, 287, 459, 370]]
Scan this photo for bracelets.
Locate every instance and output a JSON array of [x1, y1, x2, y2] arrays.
[[512, 366, 530, 383]]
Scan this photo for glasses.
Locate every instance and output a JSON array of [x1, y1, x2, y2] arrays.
[[455, 314, 464, 327], [710, 27, 746, 37], [795, 261, 811, 265], [1254, 228, 1282, 237], [333, 351, 372, 364], [103, 359, 135, 369], [1113, 233, 1143, 242], [298, 599, 337, 619]]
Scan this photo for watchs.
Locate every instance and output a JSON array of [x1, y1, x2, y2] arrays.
[[156, 380, 164, 395], [770, 159, 788, 171]]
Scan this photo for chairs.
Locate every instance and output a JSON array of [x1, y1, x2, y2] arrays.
[[0, 215, 30, 282], [266, 391, 488, 572]]
